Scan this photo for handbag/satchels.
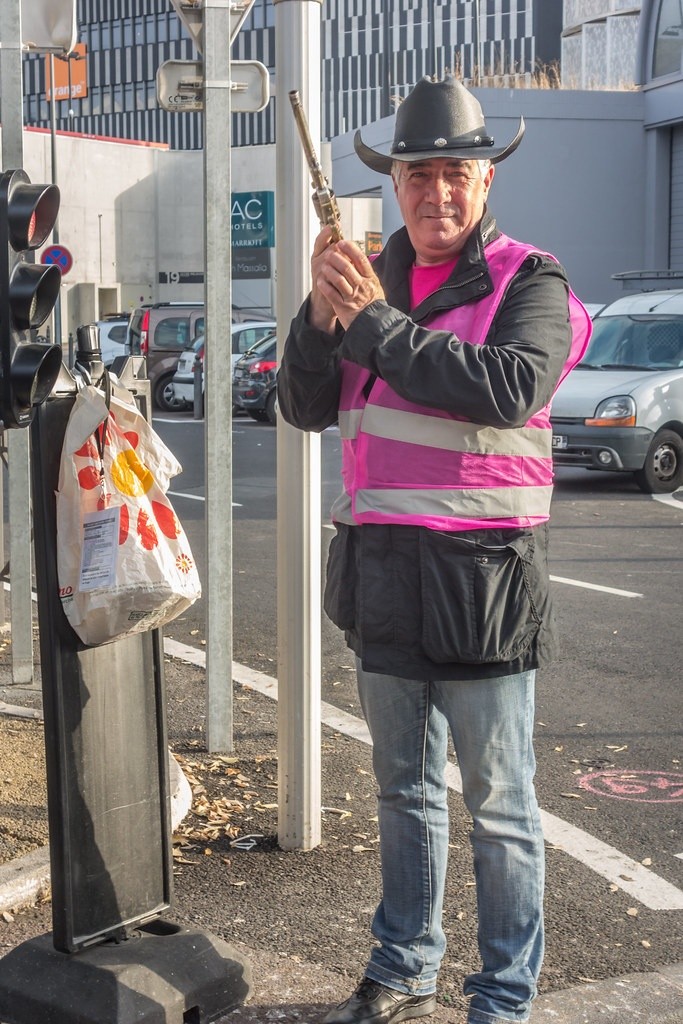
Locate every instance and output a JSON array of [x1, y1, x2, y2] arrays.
[[56, 386, 201, 647]]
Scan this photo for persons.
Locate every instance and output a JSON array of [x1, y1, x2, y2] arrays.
[[279, 78, 589, 1024]]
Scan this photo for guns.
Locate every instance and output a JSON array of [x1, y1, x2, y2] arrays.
[[286, 84, 346, 245]]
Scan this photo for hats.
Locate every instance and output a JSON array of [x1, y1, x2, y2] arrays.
[[353, 76, 524, 175]]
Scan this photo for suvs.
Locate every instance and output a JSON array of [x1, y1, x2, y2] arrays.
[[73, 299, 277, 428], [548, 288, 683, 495]]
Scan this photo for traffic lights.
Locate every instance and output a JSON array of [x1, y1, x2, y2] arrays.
[[0, 165, 64, 431]]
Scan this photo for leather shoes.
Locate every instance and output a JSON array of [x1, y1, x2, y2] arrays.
[[320, 975, 436, 1024]]
